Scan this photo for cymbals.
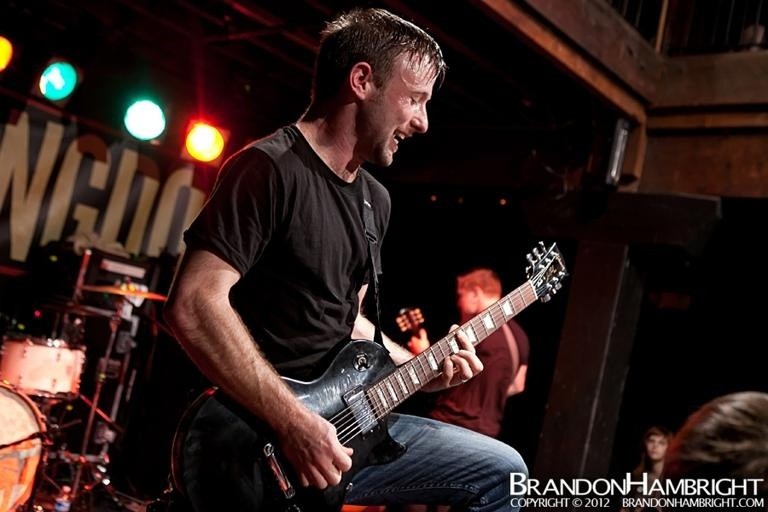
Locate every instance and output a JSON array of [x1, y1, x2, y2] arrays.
[[77, 279, 169, 303]]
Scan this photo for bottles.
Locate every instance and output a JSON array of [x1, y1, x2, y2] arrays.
[[53, 486, 71, 512]]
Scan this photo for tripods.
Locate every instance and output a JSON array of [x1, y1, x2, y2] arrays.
[[22, 308, 127, 510]]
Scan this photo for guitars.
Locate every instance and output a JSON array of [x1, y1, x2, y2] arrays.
[[170, 238, 567, 511], [396, 303, 426, 338]]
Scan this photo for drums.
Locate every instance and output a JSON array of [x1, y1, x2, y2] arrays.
[[0, 333, 86, 403], [1, 379, 52, 511]]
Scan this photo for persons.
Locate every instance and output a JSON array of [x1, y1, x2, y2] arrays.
[[658, 391, 768, 512], [407, 267, 529, 432], [628, 426, 671, 501], [160, 8, 532, 512]]
